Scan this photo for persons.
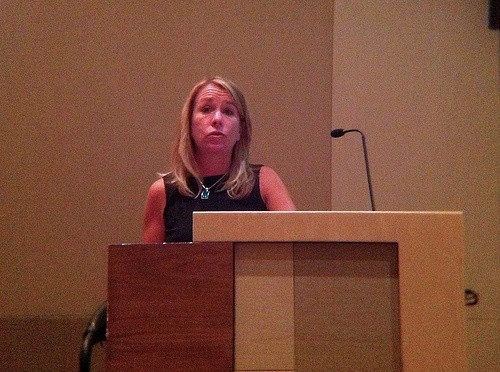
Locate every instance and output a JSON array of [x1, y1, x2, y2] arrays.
[[142, 76, 296, 243]]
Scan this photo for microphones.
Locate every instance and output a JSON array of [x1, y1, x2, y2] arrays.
[[331, 129, 375, 211]]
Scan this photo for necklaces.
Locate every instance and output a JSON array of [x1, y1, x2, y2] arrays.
[[195, 166, 231, 199]]
